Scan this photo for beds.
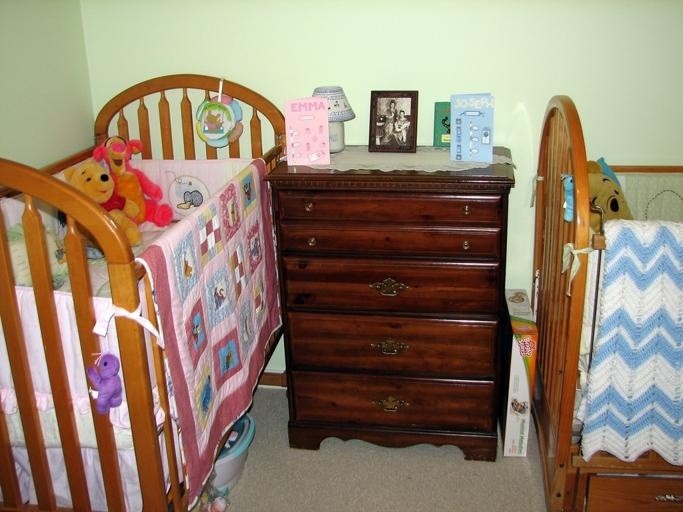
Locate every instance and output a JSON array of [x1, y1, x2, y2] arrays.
[[1, 74, 285, 512], [532, 95, 683, 512]]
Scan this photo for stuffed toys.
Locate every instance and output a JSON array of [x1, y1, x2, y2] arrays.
[[91, 135, 173, 228], [563, 176, 574, 222], [64, 156, 142, 246], [588, 160, 636, 237], [86, 352, 122, 416]]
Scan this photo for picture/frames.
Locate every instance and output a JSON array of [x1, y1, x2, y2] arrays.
[[368, 88, 419, 152]]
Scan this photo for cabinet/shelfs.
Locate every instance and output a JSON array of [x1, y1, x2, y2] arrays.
[[264, 144, 515, 462]]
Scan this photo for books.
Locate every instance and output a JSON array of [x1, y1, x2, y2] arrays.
[[285, 96, 330, 166], [434, 92, 496, 165]]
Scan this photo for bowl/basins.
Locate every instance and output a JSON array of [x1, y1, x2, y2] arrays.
[[210, 412, 255, 494]]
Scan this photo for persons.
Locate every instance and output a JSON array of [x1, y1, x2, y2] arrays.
[[380, 99, 411, 145]]
